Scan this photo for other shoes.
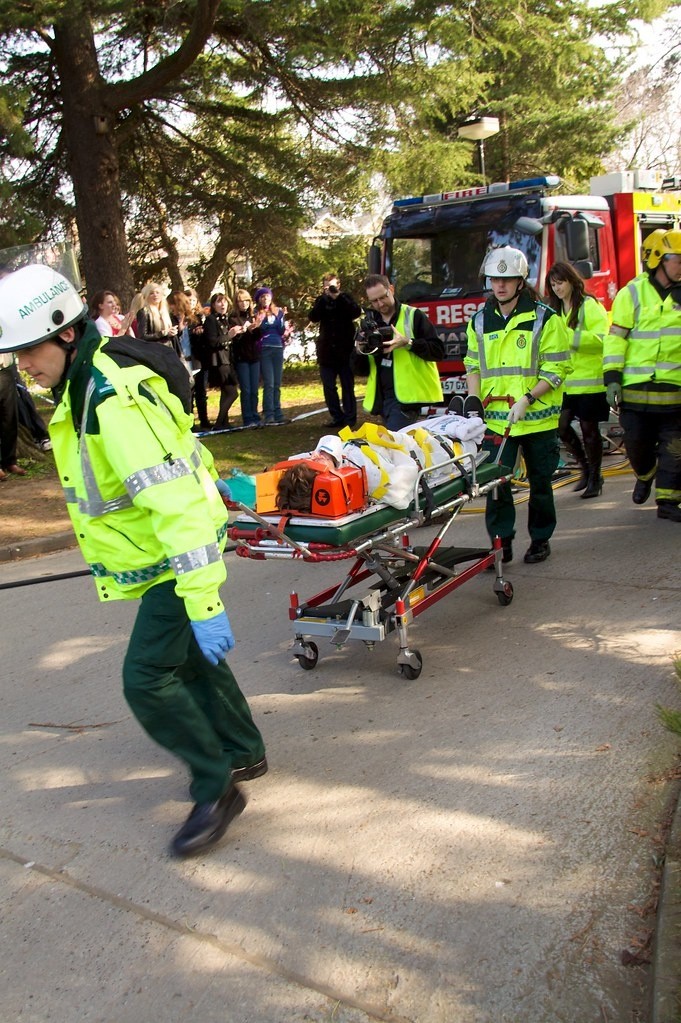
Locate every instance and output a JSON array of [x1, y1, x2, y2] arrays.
[[321, 419, 344, 428], [193, 415, 291, 432], [632, 472, 656, 504], [657, 503, 681, 522], [448, 395, 464, 416], [40, 438, 53, 452], [7, 464, 28, 476], [524, 540, 551, 562], [479, 545, 512, 570], [0, 469, 7, 480], [463, 395, 485, 424]]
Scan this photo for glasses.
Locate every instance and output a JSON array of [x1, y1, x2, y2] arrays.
[[368, 288, 389, 303]]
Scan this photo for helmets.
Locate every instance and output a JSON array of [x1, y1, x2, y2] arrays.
[[479, 245, 529, 281], [0, 264, 90, 353], [640, 229, 681, 272]]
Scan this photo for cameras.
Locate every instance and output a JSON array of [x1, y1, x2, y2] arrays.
[[359, 317, 394, 348], [329, 285, 338, 293]]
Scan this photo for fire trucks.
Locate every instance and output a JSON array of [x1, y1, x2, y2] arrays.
[[368, 169, 681, 418]]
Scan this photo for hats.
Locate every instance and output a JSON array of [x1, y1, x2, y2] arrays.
[[254, 287, 273, 300]]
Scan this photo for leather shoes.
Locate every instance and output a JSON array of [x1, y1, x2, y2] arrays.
[[228, 757, 268, 784], [170, 781, 247, 858]]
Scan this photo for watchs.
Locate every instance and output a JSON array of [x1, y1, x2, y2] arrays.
[[525, 393, 535, 405]]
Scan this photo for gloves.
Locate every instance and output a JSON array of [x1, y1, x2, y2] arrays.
[[190, 609, 234, 667], [606, 382, 622, 411], [507, 400, 530, 425], [215, 479, 231, 499]]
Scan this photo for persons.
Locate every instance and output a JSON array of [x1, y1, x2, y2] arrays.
[[308, 273, 363, 429], [0, 261, 268, 866], [544, 260, 611, 498], [602, 228, 681, 524], [0, 269, 54, 479], [94, 280, 291, 430], [463, 246, 574, 565], [358, 274, 447, 433], [274, 394, 484, 512]]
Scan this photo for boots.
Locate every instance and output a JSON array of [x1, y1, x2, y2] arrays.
[[566, 438, 589, 491], [579, 439, 604, 498]]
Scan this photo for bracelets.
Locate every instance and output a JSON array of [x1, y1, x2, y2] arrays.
[[405, 339, 412, 351]]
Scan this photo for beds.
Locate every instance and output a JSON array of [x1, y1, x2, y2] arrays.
[[224, 451, 515, 680]]
